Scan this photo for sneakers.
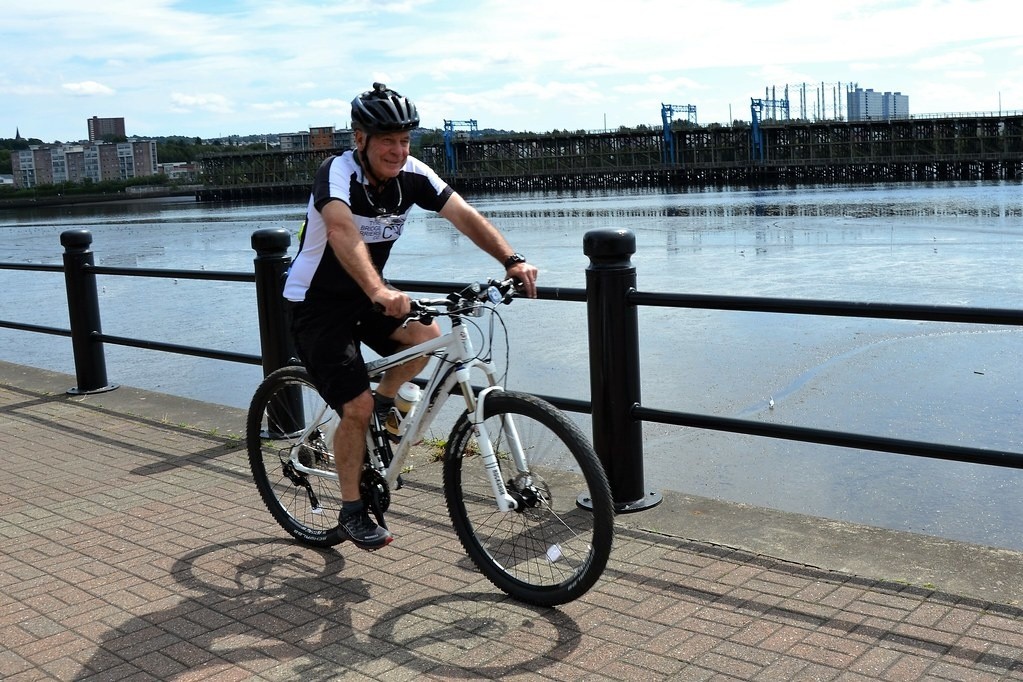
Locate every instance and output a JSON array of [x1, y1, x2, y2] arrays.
[[370, 390, 405, 445], [338, 506, 394, 550]]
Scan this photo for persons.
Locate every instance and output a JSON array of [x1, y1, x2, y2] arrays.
[[281, 82, 540, 551]]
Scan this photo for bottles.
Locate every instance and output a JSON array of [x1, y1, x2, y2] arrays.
[[385, 381, 420, 435]]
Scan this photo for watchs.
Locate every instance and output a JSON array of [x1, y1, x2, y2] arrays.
[[504, 252, 525, 271]]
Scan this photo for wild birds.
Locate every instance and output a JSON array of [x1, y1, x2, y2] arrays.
[[174, 279, 177, 282], [739, 250, 745, 253], [200, 264, 205, 267], [769, 394, 775, 410], [933, 234, 938, 251], [103, 285, 106, 290]]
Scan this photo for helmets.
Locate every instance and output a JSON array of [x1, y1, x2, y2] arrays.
[[350, 82, 419, 133]]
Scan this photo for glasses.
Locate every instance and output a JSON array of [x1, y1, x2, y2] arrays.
[[362, 179, 403, 217]]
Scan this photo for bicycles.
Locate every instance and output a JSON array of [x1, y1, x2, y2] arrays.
[[245, 277, 616, 607]]
